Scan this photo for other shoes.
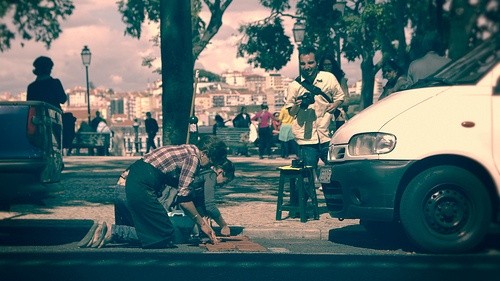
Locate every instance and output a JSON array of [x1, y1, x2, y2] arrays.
[[145, 151, 149, 154], [248, 151, 251, 155], [239, 153, 241, 156], [259, 156, 262, 159], [268, 155, 273, 159], [285, 157, 290, 159]]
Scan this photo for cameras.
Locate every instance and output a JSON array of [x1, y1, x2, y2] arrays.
[[297, 93, 315, 110]]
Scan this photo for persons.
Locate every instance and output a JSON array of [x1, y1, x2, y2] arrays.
[[91, 102, 299, 160], [25, 55, 68, 170], [408, 31, 451, 88], [377, 60, 407, 101], [77, 133, 232, 248], [286, 46, 350, 216]]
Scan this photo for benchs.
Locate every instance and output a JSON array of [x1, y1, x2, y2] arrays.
[[62, 131, 110, 156], [198, 125, 250, 157]]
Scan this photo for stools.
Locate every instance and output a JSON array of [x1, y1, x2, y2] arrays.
[[277, 165, 319, 222]]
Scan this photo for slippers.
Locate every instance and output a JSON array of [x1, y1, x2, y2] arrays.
[[78, 222, 108, 249]]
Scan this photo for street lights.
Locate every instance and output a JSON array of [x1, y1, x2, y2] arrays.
[[80, 45, 92, 124], [292, 17, 307, 77]]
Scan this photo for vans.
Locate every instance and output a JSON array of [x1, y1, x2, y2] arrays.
[[319, 30, 500, 254]]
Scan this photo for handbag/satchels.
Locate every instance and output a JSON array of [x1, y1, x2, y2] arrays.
[[335, 108, 345, 129]]
